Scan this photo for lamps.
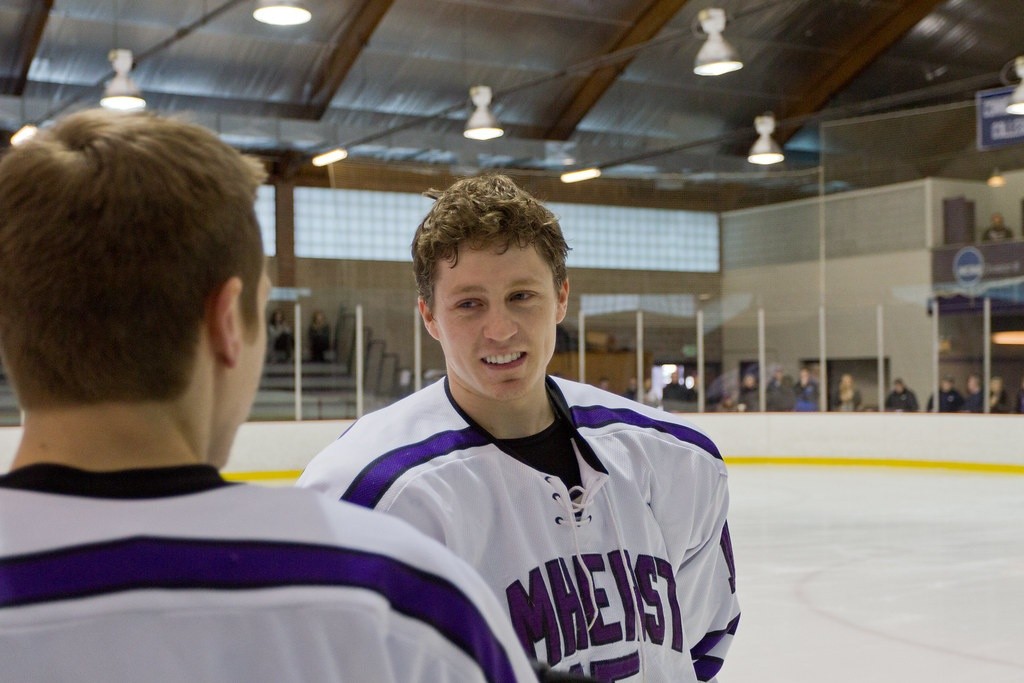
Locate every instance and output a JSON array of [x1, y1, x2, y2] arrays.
[[100, 0, 784, 183]]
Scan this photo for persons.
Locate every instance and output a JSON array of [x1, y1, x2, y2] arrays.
[[600, 376, 658, 407], [293, 173, 741, 683], [708, 373, 758, 410], [982, 213, 1012, 241], [663, 372, 698, 411], [1, 111, 538, 683], [766, 368, 818, 410], [269, 310, 294, 359], [308, 311, 330, 362], [990, 378, 1024, 413], [832, 374, 861, 412], [887, 379, 919, 411], [926, 375, 982, 411]]
